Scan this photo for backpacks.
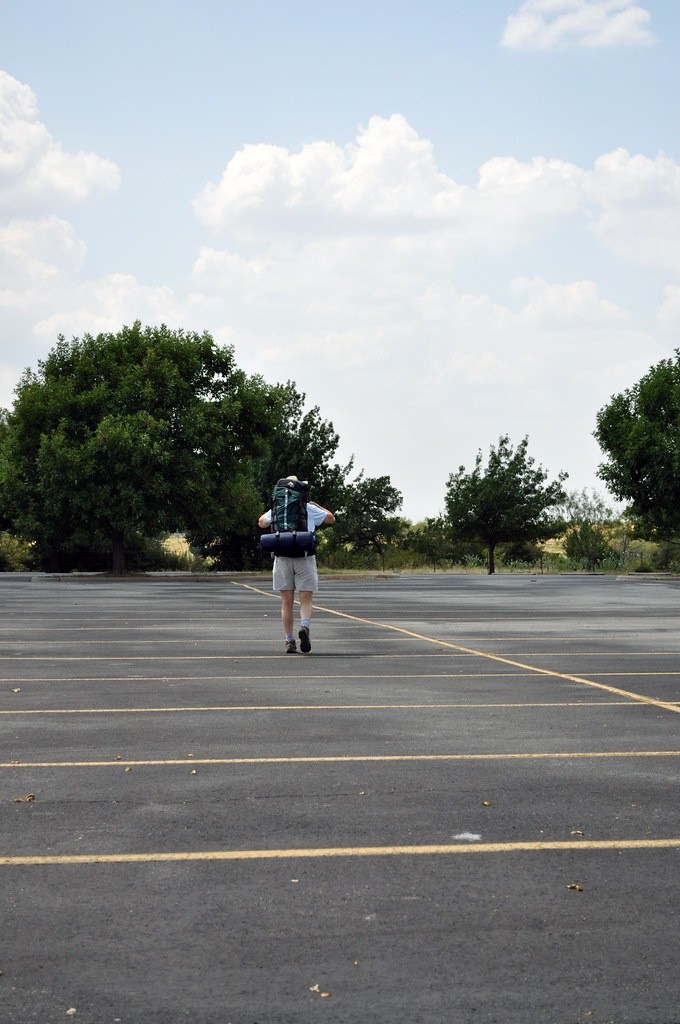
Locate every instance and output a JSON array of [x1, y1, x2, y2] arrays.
[[271, 479, 309, 531]]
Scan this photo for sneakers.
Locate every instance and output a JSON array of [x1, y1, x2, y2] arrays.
[[284, 639, 297, 654], [298, 627, 311, 653]]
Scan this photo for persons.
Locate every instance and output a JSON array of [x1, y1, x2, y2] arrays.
[[258, 476, 335, 654]]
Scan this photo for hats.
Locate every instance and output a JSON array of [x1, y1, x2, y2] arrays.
[[285, 475, 307, 484]]
